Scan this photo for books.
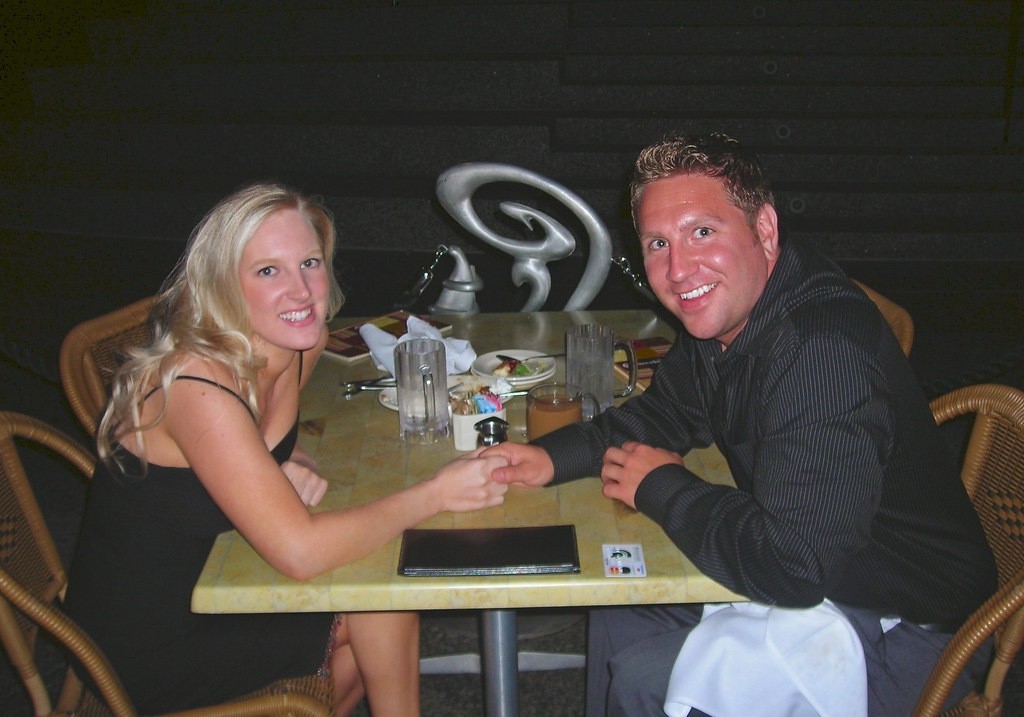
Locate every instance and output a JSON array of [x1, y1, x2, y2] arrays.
[[611, 336, 672, 392], [323, 310, 453, 363]]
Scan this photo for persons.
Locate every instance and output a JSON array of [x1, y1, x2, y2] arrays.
[[479, 130, 999, 716], [43, 182, 511, 717]]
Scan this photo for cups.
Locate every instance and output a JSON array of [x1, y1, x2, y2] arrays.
[[525, 384, 601, 442], [567, 325, 638, 417], [452, 408, 507, 452]]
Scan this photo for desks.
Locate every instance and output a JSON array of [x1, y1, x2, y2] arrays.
[[192, 310, 753, 717]]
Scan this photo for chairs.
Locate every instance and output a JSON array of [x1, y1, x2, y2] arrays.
[[0, 294, 338, 717], [848, 277, 1024, 717]]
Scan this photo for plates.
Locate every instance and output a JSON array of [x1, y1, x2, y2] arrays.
[[394, 339, 451, 444], [469, 349, 555, 380], [470, 368, 556, 385], [446, 375, 513, 403], [378, 390, 398, 411]]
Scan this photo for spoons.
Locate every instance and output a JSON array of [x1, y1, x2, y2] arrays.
[[496, 355, 566, 363]]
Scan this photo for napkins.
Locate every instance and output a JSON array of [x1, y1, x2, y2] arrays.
[[663, 595, 868, 717], [359, 314, 477, 381]]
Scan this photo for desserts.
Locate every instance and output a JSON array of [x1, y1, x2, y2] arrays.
[[448, 386, 507, 451]]
